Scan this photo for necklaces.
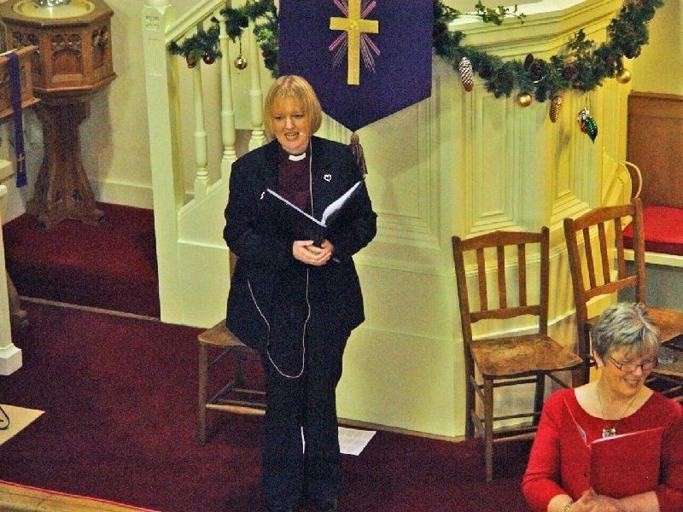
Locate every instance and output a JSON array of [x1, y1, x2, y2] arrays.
[[597, 380, 641, 440]]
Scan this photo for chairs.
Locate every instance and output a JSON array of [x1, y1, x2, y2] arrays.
[[564, 198, 683, 401], [451, 226, 584, 482], [197, 248, 266, 446]]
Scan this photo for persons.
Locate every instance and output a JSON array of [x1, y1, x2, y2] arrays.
[[521, 299, 682, 512], [222, 74, 379, 511]]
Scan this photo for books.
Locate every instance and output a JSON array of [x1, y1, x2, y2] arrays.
[[558, 397, 661, 501], [258, 181, 362, 249]]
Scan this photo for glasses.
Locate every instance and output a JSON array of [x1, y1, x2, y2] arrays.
[[608, 354, 658, 374]]
